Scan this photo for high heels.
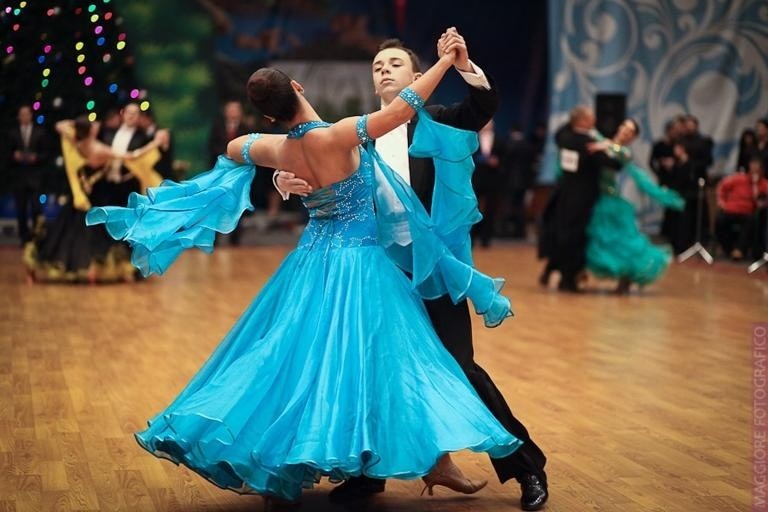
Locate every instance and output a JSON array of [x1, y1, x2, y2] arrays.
[[423, 458, 490, 498]]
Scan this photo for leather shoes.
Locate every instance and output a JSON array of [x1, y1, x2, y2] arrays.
[[515, 464, 550, 511], [327, 473, 387, 501]]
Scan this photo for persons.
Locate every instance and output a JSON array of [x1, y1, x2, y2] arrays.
[[270, 27, 547, 511], [225, 27, 491, 510], [6, 98, 181, 288], [208, 98, 257, 248]]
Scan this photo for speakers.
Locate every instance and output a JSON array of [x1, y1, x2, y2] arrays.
[[595, 90, 625, 139]]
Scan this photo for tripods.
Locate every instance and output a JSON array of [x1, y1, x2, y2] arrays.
[[677, 178, 713, 264]]
